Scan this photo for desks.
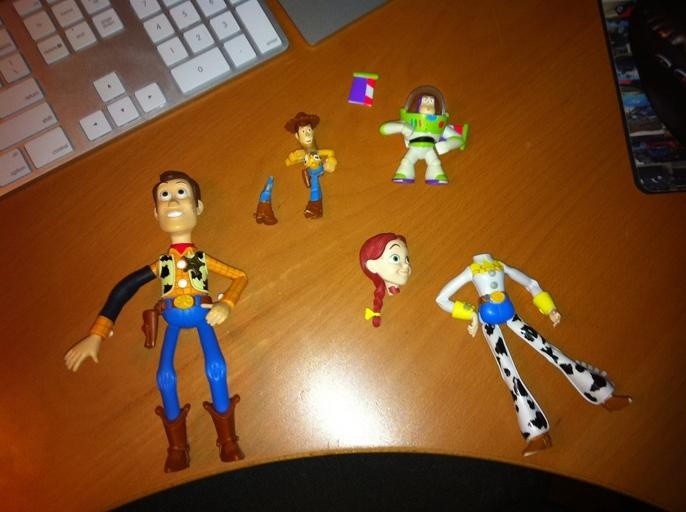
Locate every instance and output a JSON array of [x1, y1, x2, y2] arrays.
[[0, 3, 686, 512]]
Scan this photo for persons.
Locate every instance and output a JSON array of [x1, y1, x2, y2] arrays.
[[254, 112, 337, 226], [380, 85, 469, 186], [359, 231, 634, 459], [63, 170, 248, 474]]
